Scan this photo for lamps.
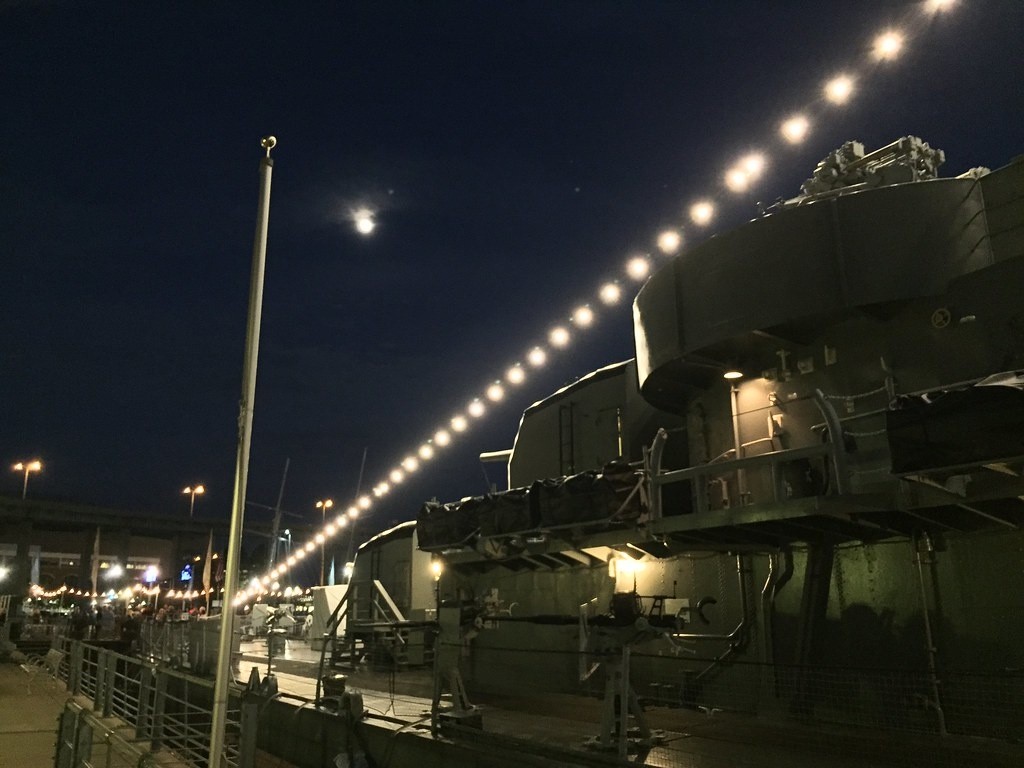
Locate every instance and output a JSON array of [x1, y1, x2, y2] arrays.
[[721, 365, 743, 378]]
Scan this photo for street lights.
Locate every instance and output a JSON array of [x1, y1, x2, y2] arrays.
[[316, 498, 335, 588], [12, 458, 42, 501], [182, 485, 205, 518], [203, 135, 277, 768]]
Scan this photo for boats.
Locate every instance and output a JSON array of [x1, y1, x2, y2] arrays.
[[133, 129, 1024, 768]]
